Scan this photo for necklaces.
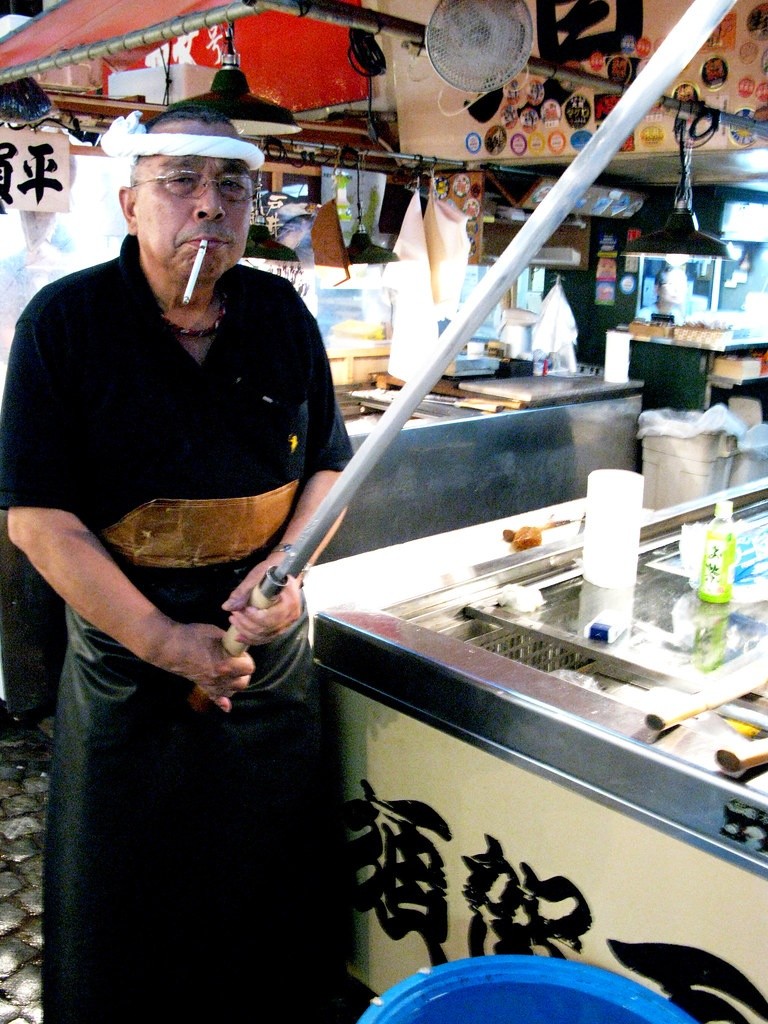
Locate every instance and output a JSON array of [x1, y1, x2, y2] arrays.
[[162, 296, 225, 338]]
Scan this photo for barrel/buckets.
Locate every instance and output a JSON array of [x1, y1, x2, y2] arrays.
[[356, 954, 702, 1024]]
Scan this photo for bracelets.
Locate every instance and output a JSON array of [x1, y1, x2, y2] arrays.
[[273, 542, 310, 589]]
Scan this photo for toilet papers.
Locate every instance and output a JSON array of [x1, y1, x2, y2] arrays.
[[603, 329, 630, 384], [579, 468, 646, 592]]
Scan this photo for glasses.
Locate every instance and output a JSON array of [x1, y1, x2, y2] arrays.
[[129, 168, 261, 201]]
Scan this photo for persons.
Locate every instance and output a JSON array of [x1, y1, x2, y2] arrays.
[[2, 104, 355, 1023], [635, 265, 689, 326]]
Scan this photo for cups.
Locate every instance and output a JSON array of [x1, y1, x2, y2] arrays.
[[603, 329, 633, 382], [582, 470, 645, 588]]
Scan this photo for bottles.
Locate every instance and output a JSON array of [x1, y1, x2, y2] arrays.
[[698, 501, 736, 604]]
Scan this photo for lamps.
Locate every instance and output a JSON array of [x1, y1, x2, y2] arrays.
[[344, 162, 401, 264], [615, 95, 734, 262], [241, 170, 299, 264], [167, 21, 304, 138]]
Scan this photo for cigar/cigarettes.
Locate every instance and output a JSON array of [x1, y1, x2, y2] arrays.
[[181, 238, 209, 306]]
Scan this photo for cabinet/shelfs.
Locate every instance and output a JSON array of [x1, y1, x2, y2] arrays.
[[627, 340, 768, 412]]
